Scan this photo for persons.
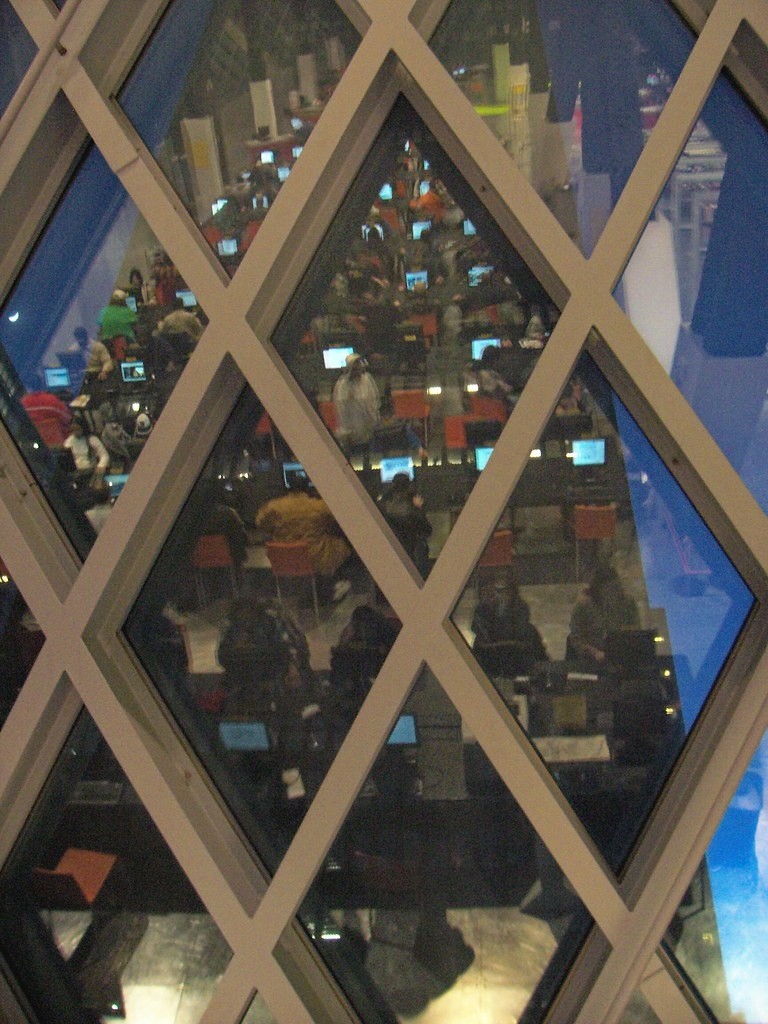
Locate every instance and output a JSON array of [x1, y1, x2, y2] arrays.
[[568, 564, 641, 663], [471, 582, 548, 676], [218, 595, 310, 696], [329, 603, 399, 695], [20, 73, 623, 594]]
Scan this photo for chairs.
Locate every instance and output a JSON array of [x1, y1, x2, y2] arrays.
[[1, 181, 617, 901]]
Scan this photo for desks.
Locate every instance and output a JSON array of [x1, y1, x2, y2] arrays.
[[24, 68, 680, 813]]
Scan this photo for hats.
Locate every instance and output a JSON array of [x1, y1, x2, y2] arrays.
[[113, 289, 129, 300]]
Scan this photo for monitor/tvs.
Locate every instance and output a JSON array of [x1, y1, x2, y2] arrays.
[[41, 116, 672, 766]]
